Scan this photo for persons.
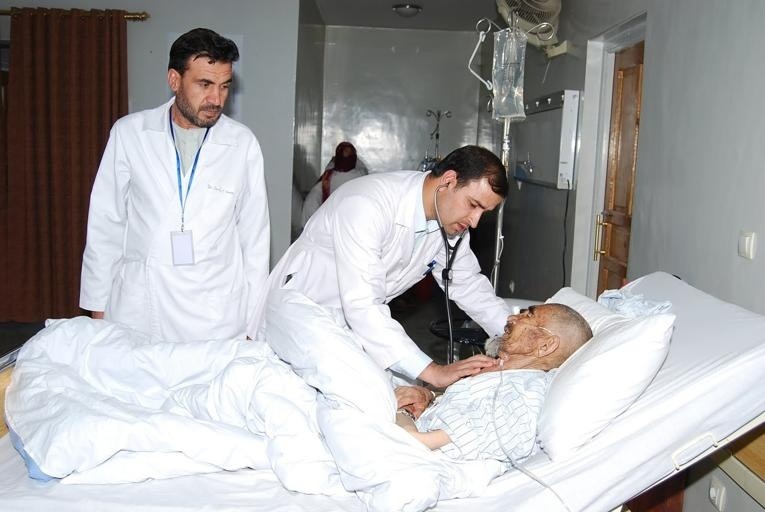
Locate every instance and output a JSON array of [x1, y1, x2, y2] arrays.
[[77, 27, 273, 344], [391, 302, 596, 484], [243, 145, 523, 389], [303, 141, 369, 231]]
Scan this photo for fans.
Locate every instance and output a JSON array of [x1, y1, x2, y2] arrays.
[[495, 0, 568, 58]]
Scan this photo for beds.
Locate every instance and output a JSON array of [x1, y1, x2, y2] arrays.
[[1, 271, 765, 512]]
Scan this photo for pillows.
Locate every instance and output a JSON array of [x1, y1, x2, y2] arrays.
[[499, 287, 676, 460]]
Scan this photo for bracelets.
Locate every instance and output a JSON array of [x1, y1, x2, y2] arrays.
[[396, 406, 418, 423]]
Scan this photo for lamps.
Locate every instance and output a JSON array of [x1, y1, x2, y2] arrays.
[[392, 4, 423, 17]]
